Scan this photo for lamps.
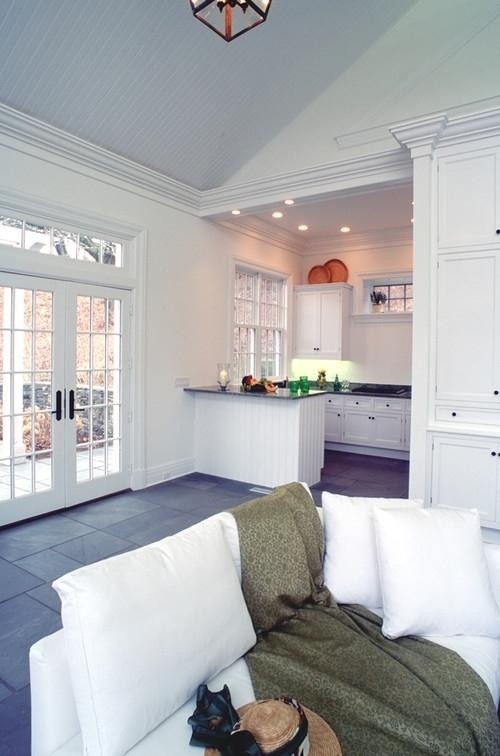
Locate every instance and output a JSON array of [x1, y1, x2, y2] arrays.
[[190, 0, 272, 43]]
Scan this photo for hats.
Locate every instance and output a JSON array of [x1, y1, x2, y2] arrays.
[[203, 698, 342, 756]]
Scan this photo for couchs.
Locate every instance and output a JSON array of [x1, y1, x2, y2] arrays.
[[28, 506, 500, 756]]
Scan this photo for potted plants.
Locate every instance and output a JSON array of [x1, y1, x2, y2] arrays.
[[369, 291, 388, 313]]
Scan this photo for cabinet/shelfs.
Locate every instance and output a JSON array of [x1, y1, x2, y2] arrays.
[[291, 281, 354, 360], [325, 393, 411, 462], [388, 107, 500, 545]]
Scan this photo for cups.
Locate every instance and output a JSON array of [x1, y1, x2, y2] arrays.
[[372, 304, 383, 313], [290, 381, 299, 392], [301, 382, 308, 393], [300, 376, 307, 390]]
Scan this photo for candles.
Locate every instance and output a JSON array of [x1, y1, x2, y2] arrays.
[[219, 369, 227, 385]]
